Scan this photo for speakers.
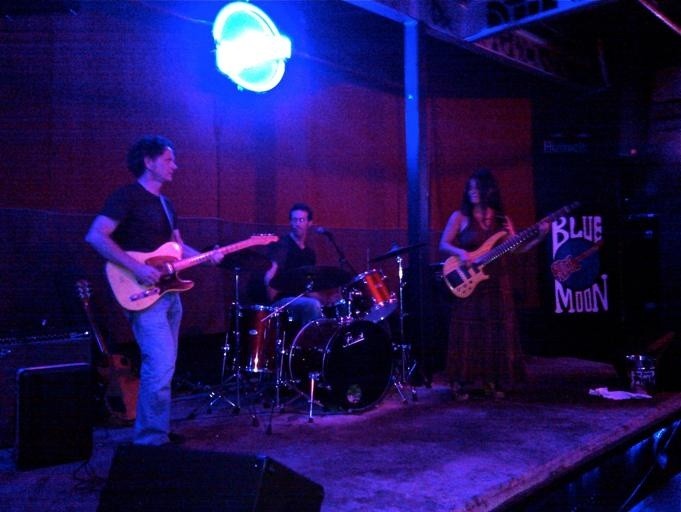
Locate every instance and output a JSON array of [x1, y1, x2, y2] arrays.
[[95, 441, 323, 512]]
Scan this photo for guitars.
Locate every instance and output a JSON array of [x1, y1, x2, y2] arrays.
[[442, 203, 578, 300], [76, 279, 140, 426], [107, 234, 279, 311]]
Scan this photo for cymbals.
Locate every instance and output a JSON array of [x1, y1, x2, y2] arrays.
[[269, 265, 352, 292], [201, 244, 271, 272], [374, 242, 429, 261]]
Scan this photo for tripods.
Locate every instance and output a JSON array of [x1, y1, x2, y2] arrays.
[[387, 270, 419, 404], [188, 274, 317, 436]]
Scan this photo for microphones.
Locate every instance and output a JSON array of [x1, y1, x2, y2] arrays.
[[315, 227, 332, 237]]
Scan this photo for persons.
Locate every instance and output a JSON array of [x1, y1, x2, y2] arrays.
[[262, 201, 318, 299], [85, 132, 225, 445], [439, 167, 549, 406]]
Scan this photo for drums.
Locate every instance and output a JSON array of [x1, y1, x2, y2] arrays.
[[289, 318, 393, 414], [342, 267, 399, 323], [320, 299, 359, 318], [236, 304, 282, 373]]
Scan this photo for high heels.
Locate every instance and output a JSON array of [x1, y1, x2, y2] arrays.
[[451, 383, 469, 402], [485, 384, 500, 402]]
